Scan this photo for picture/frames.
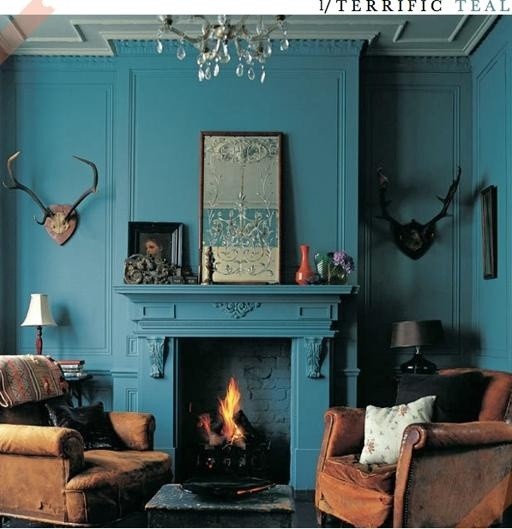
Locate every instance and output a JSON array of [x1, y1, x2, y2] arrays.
[[482, 185, 497, 280], [127, 223, 182, 268]]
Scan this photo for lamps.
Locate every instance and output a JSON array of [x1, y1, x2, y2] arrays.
[[391, 321, 446, 372], [22, 294, 58, 355], [155, 11, 290, 83]]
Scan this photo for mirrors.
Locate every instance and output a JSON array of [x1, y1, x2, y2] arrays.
[[198, 131, 282, 283]]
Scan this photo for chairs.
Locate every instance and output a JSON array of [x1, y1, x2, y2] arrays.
[[313, 370, 511, 528], [2, 356, 174, 528]]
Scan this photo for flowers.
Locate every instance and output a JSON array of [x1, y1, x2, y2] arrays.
[[314, 251, 355, 279]]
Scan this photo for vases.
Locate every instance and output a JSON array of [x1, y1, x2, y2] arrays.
[[323, 276, 343, 285], [295, 245, 313, 285]]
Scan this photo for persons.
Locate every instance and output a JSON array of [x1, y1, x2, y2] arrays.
[[143, 235, 166, 263]]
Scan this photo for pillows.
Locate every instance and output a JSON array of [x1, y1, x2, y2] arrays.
[[41, 402, 116, 451], [359, 371, 487, 464]]
